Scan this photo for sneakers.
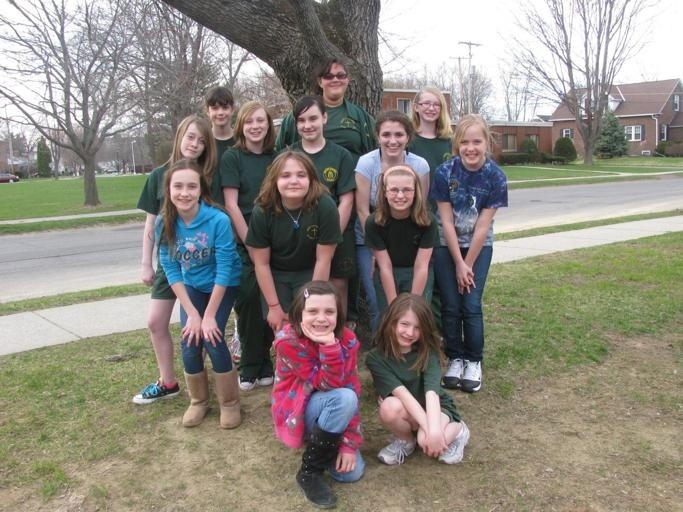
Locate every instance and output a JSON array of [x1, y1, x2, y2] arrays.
[[462, 361, 482, 392], [438, 417, 470, 464], [377, 437, 416, 465], [237, 358, 274, 389], [441, 357, 463, 392], [230, 329, 242, 363], [131, 379, 180, 404]]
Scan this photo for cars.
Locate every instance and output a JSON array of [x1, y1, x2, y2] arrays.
[[0, 159, 121, 184]]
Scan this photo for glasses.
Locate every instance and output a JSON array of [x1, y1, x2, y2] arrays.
[[323, 73, 347, 80]]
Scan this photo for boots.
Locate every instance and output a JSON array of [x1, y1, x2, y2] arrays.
[[296, 418, 343, 509], [212, 368, 241, 429], [181, 367, 210, 427]]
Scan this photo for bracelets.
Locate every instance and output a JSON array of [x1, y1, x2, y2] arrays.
[[267, 303, 280, 307]]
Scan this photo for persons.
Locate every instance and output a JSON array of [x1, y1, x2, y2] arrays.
[[354, 111, 431, 333], [270, 280, 364, 507], [131, 114, 224, 405], [219, 100, 277, 391], [152, 157, 244, 430], [428, 113, 509, 396], [276, 96, 358, 324], [406, 87, 456, 331], [245, 150, 343, 335], [275, 59, 380, 321], [365, 292, 470, 466], [203, 86, 243, 364], [364, 164, 440, 321]]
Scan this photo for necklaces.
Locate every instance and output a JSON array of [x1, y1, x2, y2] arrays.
[[283, 206, 302, 230]]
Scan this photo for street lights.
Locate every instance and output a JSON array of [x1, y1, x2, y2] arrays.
[[130, 139, 140, 174]]
